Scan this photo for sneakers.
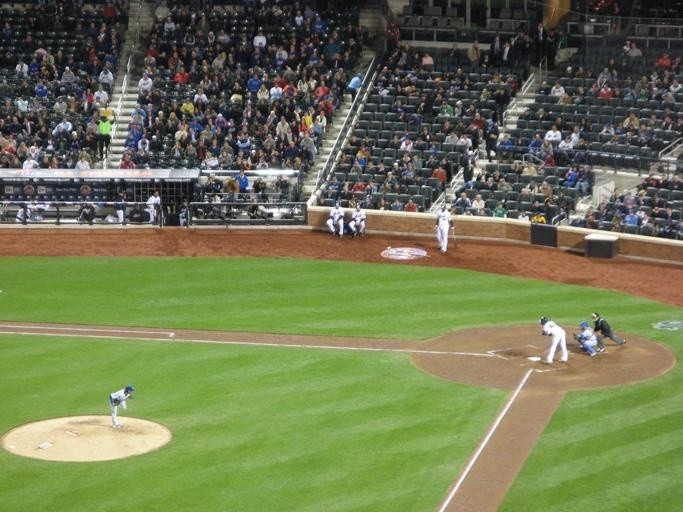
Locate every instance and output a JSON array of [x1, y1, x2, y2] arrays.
[[595, 347, 604, 352], [590, 352, 597, 357]]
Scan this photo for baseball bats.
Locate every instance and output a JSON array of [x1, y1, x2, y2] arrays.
[[452, 229, 458, 248]]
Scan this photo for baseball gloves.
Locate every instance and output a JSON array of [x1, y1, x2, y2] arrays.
[[572, 333, 579, 340]]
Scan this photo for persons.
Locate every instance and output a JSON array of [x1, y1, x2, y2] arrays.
[[109, 385, 134, 429], [1, 0, 681, 242], [588, 312, 625, 352], [574, 321, 598, 358], [538, 316, 568, 365], [433, 203, 454, 255]]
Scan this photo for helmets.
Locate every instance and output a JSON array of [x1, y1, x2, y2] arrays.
[[591, 312, 599, 322], [540, 316, 547, 325], [579, 321, 588, 330]]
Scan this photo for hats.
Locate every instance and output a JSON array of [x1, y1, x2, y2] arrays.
[[126, 386, 134, 392]]
